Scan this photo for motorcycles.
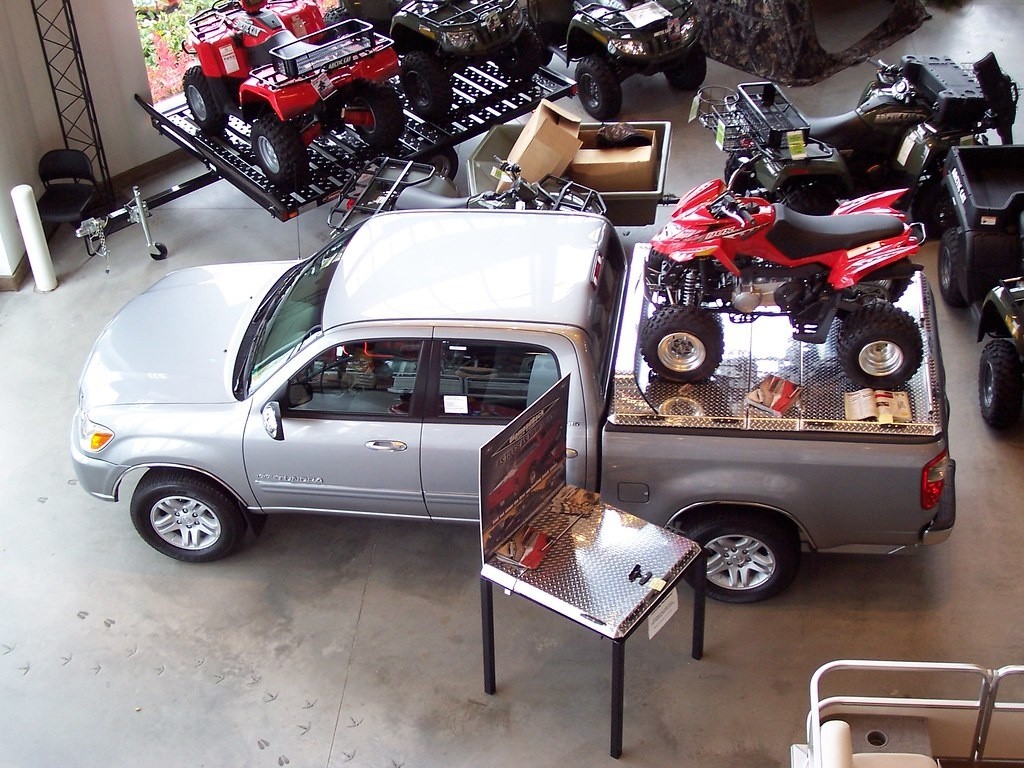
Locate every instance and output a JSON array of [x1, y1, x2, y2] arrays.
[[693, 51, 1024, 216], [179, 1, 407, 192], [321, 1, 543, 119], [909, 145, 1023, 425], [516, 0, 708, 122], [642, 154, 930, 386], [318, 159, 610, 242]]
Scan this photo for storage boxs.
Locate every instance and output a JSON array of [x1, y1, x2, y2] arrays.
[[558, 129, 658, 192], [496, 100, 584, 194]]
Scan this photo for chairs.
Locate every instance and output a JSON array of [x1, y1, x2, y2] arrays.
[[481, 377, 707, 761], [37, 150, 113, 258]]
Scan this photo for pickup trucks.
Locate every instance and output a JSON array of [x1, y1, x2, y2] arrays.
[[71, 205, 959, 603]]
